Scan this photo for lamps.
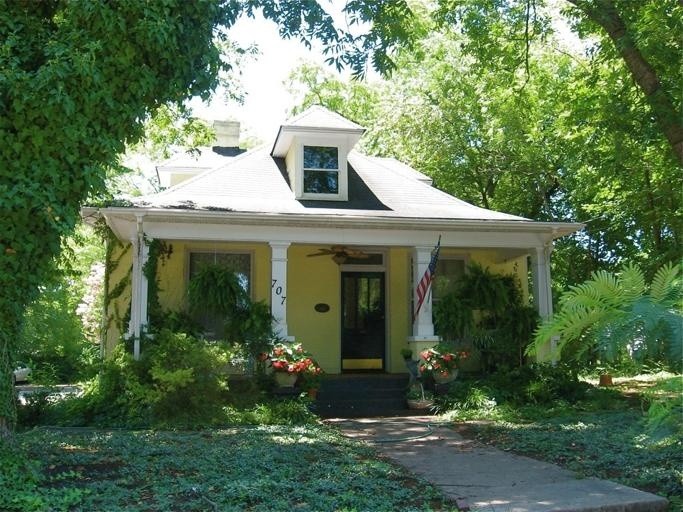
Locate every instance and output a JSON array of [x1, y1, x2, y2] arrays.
[[332, 251, 348, 266]]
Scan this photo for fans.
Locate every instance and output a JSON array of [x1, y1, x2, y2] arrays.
[[307, 245, 370, 267]]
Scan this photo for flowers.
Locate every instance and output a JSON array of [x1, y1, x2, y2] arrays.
[[415, 344, 470, 378], [256, 340, 324, 386], [407, 384, 434, 400]]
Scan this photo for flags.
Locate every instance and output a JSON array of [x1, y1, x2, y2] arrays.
[[416, 241, 440, 312]]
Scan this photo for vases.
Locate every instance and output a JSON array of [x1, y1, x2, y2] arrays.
[[434, 370, 458, 384], [408, 400, 432, 410], [598, 374, 612, 385], [272, 370, 318, 402]]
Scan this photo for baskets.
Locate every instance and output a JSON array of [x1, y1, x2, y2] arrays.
[[406, 383, 435, 410]]
